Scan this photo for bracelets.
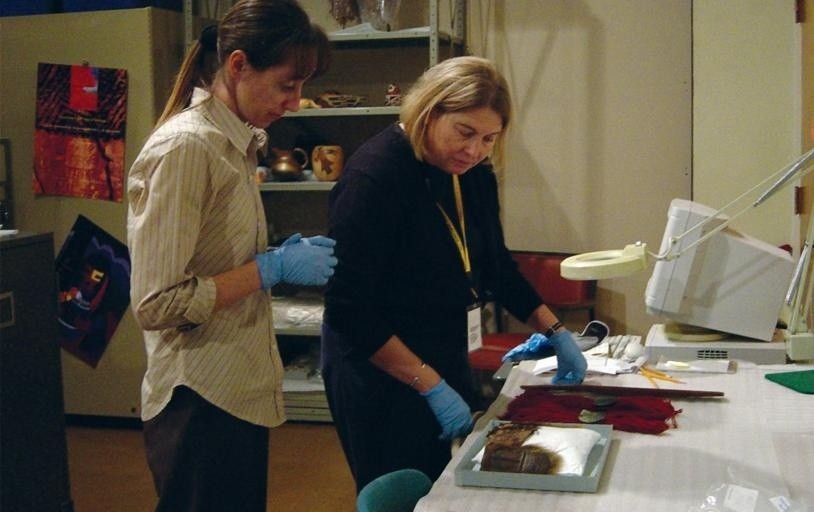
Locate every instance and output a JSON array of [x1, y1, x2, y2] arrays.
[[540, 318, 564, 338], [406, 358, 428, 393]]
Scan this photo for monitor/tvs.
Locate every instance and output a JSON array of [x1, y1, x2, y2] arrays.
[[644, 198, 795, 341]]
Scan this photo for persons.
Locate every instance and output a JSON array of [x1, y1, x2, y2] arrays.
[[315, 53, 588, 511], [122, 0, 340, 511]]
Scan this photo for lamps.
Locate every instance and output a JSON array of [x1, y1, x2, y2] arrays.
[[555, 141, 813, 366]]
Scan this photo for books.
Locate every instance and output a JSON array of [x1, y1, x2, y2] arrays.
[[273, 357, 339, 422]]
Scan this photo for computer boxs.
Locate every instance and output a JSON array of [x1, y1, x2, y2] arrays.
[[644, 324, 788, 365]]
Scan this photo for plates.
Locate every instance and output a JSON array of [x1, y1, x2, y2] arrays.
[[320, 94, 367, 107]]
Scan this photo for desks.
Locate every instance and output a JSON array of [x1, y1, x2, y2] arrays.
[[412, 338, 814, 512]]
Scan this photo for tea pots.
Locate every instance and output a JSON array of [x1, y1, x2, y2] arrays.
[[271, 146, 309, 179]]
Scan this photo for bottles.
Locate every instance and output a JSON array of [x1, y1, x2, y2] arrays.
[[382, 81, 400, 106]]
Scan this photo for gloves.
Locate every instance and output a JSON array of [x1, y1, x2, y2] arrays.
[[419, 379, 474, 441], [255, 238, 338, 291], [266, 232, 337, 254], [549, 330, 588, 384]]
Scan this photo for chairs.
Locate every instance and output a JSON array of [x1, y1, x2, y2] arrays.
[[350, 470, 428, 512], [465, 252, 598, 384]]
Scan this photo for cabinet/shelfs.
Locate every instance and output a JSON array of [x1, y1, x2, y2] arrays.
[[184, 2, 468, 429], [1, 230, 75, 512]]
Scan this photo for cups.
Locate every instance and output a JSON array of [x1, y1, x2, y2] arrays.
[[311, 145, 344, 181], [374, 0, 400, 33]]
